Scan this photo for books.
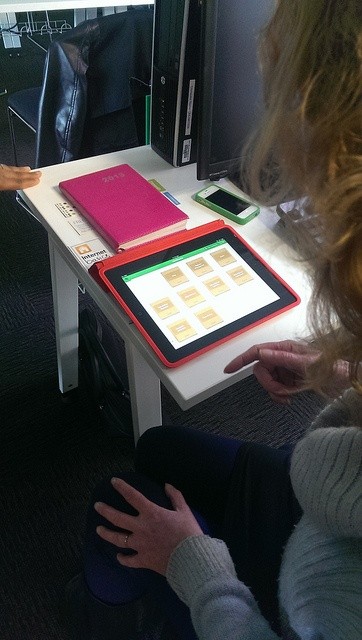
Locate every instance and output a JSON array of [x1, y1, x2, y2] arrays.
[[59, 164, 189, 254]]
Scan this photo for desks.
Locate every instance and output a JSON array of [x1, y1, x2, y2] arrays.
[[13, 147, 342, 448]]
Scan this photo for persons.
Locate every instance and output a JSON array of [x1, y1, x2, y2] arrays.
[[0, 163, 42, 191], [95, 1, 361, 639]]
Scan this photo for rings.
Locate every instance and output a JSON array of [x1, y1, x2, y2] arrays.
[[124, 536, 129, 548]]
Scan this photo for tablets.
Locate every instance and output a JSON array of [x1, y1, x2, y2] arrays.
[[88, 218, 300, 368]]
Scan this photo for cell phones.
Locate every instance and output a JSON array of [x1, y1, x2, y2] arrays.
[[191, 182, 259, 225]]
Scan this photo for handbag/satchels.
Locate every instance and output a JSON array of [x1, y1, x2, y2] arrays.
[[78, 301, 135, 452]]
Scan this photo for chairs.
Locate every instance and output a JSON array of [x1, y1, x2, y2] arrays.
[[6, 10, 154, 161]]
